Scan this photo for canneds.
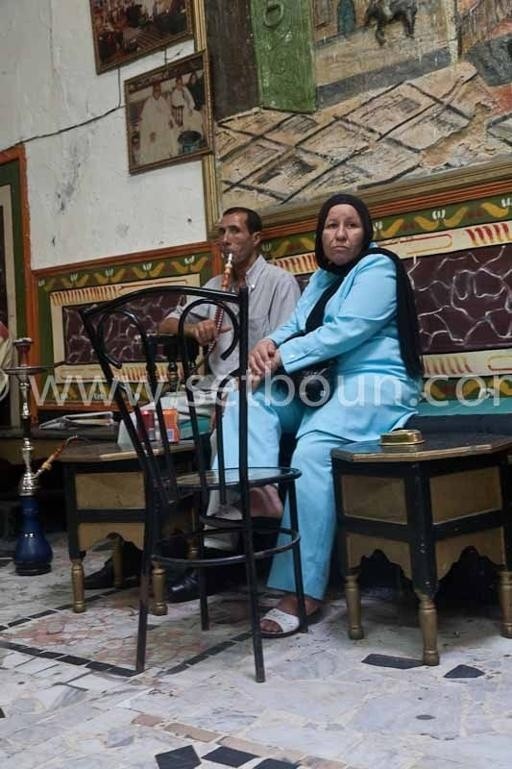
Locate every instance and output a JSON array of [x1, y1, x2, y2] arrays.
[[135, 410, 155, 441], [159, 408, 181, 444]]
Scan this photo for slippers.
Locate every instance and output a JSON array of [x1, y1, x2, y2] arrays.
[[260, 608, 322, 638], [198, 504, 282, 529]]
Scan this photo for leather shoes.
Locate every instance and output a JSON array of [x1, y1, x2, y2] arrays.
[[84, 541, 243, 603]]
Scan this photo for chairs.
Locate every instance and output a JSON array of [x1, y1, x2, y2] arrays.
[[75, 285, 308, 684]]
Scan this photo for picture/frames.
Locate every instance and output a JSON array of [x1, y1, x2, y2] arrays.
[[89, 0, 194, 76], [125, 49, 214, 174]]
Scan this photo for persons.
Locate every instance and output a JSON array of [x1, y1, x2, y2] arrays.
[[138, 81, 178, 165], [157, 203, 307, 602], [212, 194, 426, 641], [169, 77, 204, 141], [186, 73, 206, 112]]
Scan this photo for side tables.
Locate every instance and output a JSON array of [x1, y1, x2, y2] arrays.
[[331, 434, 511, 666], [56, 439, 200, 615]]
